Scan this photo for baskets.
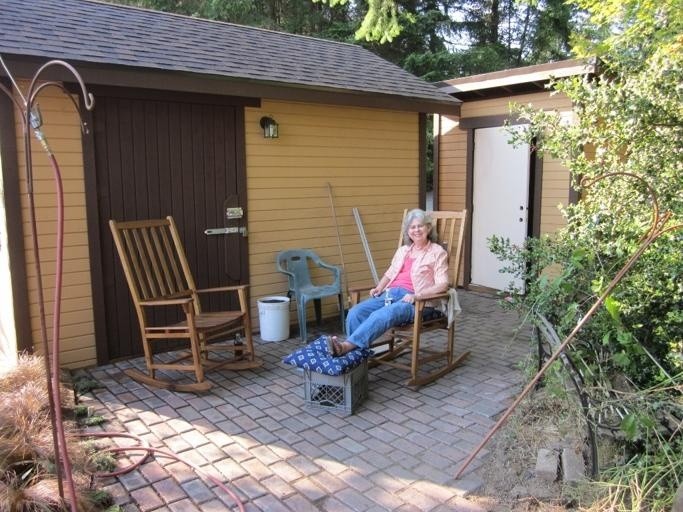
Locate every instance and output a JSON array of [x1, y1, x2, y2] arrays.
[[302, 358, 368, 415]]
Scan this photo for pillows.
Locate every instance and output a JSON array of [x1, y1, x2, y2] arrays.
[[284, 334, 375, 377]]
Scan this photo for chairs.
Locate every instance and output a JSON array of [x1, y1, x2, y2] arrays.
[[108, 215, 267, 393], [343, 206, 472, 387], [276, 249, 346, 344]]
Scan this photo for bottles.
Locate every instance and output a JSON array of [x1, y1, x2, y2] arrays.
[[384, 287, 394, 306], [233, 333, 245, 361]]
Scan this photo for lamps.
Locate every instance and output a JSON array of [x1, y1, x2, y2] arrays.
[[260, 116, 279, 138]]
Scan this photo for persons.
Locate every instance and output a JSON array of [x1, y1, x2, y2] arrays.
[[326, 208, 449, 359]]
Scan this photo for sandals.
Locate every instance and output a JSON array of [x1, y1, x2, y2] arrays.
[[326, 334, 345, 358]]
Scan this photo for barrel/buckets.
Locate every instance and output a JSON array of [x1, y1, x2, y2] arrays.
[[256, 295, 291, 342]]
